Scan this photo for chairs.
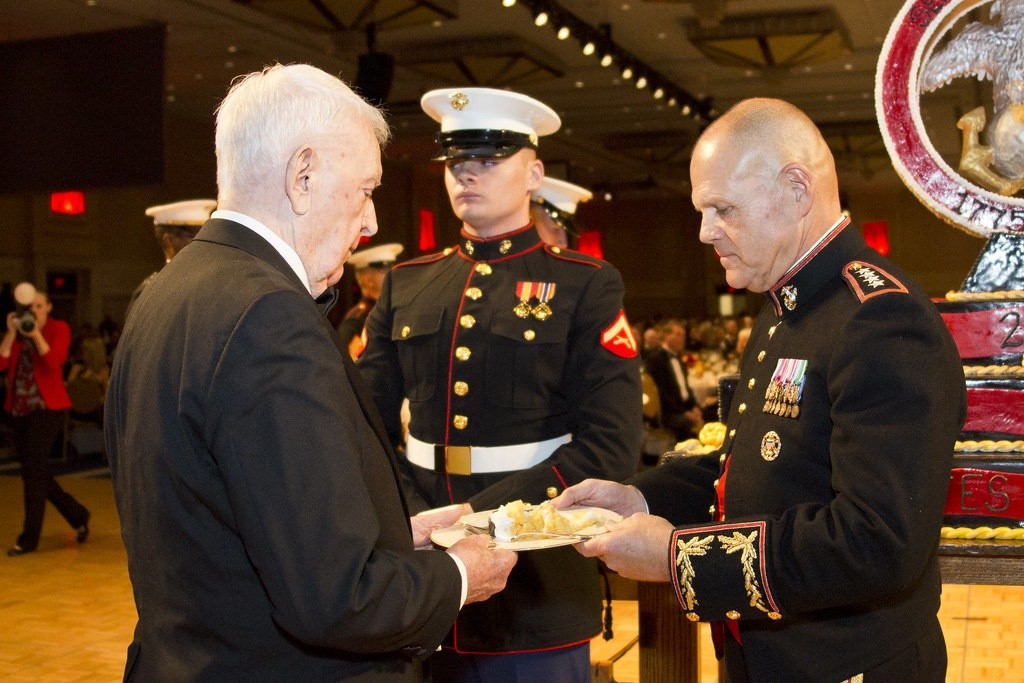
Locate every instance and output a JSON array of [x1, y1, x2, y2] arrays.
[[638, 373, 677, 466], [62, 376, 108, 467]]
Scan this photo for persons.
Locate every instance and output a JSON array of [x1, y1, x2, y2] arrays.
[[105, 65, 519, 683], [356, 88, 644, 683], [125, 199, 218, 318], [551, 98, 968, 683], [634, 315, 753, 441], [0, 291, 90, 557], [338, 243, 404, 363], [529, 176, 593, 248]]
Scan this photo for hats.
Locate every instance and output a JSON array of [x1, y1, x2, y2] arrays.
[[530, 175, 593, 233], [145, 199, 218, 236], [347, 244, 404, 271], [421, 87, 560, 162]]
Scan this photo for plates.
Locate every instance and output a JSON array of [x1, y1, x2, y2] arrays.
[[430, 505, 624, 551]]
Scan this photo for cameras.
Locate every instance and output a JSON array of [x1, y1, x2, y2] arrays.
[[11, 283, 36, 333]]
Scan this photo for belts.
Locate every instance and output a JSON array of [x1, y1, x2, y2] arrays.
[[404, 433, 572, 475]]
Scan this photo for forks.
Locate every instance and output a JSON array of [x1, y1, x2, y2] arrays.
[[465, 524, 596, 542]]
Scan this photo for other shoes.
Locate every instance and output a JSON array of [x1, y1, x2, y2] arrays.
[[7, 546, 38, 556], [76, 513, 92, 543]]
